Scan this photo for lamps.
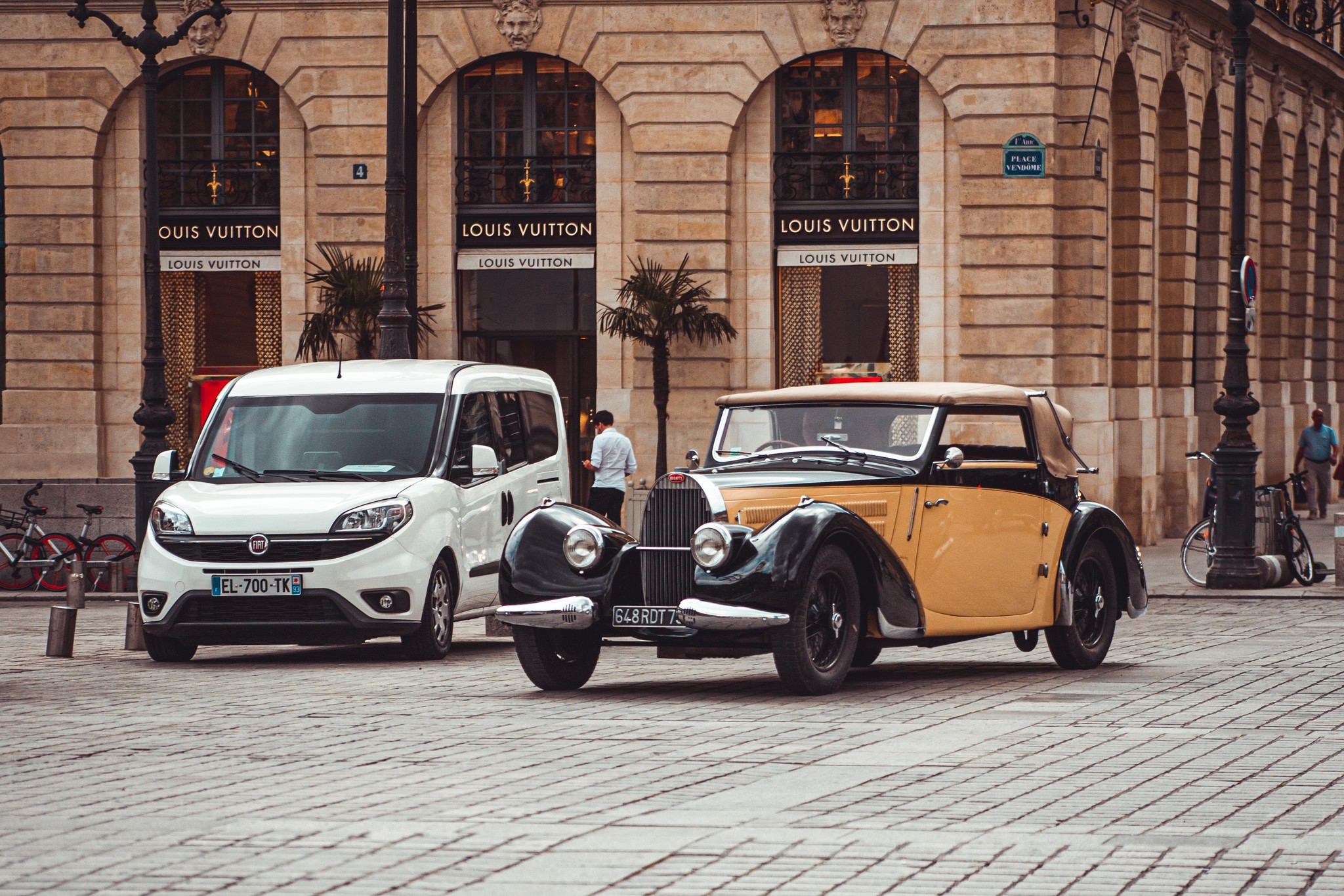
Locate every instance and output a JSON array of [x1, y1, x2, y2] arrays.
[[806, 105, 844, 151]]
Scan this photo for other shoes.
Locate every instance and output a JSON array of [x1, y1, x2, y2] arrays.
[[1319, 508, 1327, 518], [1307, 513, 1318, 520]]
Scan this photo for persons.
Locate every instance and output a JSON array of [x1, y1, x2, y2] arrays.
[[1293, 409, 1339, 521], [1332, 444, 1344, 500], [492, 0, 543, 51], [820, 0, 866, 48], [176, 1, 226, 55], [583, 409, 637, 528]]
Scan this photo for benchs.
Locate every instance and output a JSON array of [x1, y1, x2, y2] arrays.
[[886, 443, 1029, 462]]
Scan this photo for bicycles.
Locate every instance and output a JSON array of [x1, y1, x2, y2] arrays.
[[1254, 468, 1316, 587], [1178, 450, 1215, 587], [0, 482, 138, 592]]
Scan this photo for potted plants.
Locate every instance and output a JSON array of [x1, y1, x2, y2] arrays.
[[594, 250, 740, 543]]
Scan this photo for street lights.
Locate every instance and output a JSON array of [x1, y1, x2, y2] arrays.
[[67, 0, 231, 601]]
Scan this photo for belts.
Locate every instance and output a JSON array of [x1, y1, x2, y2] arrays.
[[1305, 457, 1331, 464]]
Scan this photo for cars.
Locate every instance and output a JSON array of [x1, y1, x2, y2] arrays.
[[496, 381, 1149, 691]]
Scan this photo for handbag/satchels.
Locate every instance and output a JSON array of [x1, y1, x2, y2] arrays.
[[1291, 477, 1308, 504]]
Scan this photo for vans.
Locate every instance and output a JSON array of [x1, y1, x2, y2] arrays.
[[138, 359, 571, 665]]
